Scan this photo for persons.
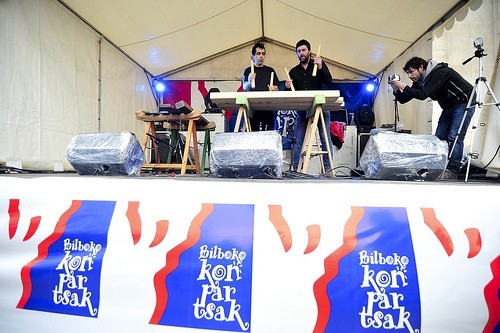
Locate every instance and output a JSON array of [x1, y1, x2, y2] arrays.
[[388, 56, 477, 181], [241, 44, 282, 132], [285, 39, 334, 177]]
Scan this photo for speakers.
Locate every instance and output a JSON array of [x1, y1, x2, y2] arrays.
[[359, 132, 448, 181], [66, 131, 147, 177], [211, 131, 282, 178]]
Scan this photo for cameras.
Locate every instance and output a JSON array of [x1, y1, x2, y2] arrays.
[[388, 72, 400, 83]]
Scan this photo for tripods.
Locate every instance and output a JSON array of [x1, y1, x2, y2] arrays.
[[439, 54, 500, 183]]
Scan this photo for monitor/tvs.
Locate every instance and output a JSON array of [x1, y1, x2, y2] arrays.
[[329, 109, 348, 125]]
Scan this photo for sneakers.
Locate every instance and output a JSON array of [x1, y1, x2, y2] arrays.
[[437, 172, 458, 179]]
